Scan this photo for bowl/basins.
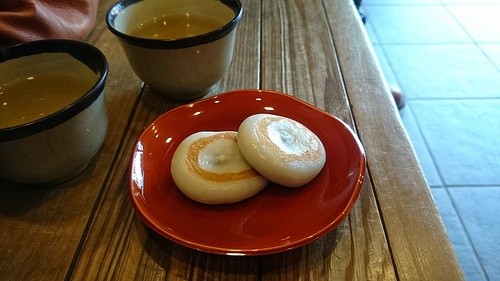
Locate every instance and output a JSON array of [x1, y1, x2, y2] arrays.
[[0, 39, 109, 187], [105, 0, 243, 100]]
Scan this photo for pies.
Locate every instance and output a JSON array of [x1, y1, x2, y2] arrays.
[[237, 114, 326, 187], [170, 131, 269, 205]]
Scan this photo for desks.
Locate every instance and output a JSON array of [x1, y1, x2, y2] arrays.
[[0, 0, 465, 281]]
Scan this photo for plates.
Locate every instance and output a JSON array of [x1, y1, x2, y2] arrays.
[[128, 90, 366, 256]]
